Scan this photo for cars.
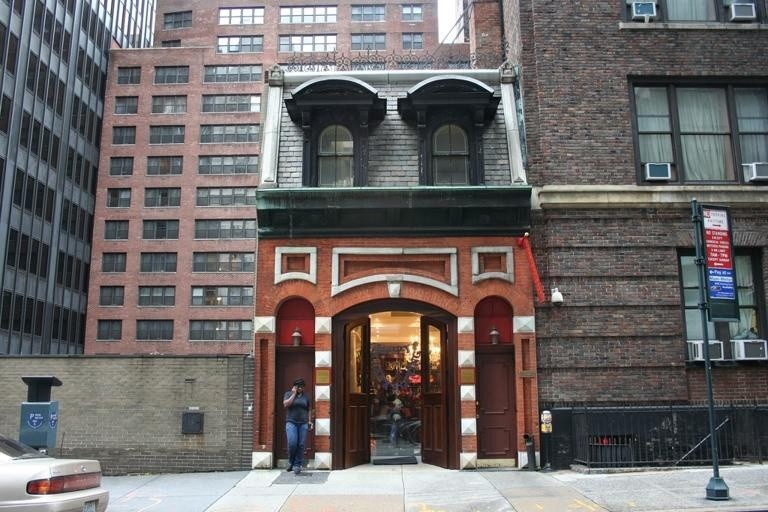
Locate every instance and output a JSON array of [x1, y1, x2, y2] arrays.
[[0, 434, 110, 512]]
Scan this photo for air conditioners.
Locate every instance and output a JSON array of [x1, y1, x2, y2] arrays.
[[645, 163, 671, 180], [749, 162, 768, 181], [729, 3, 756, 20], [686, 340, 768, 361], [631, 1, 657, 19]]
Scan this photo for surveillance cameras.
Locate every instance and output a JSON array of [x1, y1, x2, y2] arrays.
[[551, 288, 563, 306]]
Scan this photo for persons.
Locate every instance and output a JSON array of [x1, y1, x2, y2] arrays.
[[283, 378, 314, 474]]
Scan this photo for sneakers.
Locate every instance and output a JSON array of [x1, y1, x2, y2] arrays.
[[286, 463, 300, 475]]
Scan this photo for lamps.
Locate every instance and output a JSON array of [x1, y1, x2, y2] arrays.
[[488, 324, 501, 345], [291, 326, 303, 345]]
